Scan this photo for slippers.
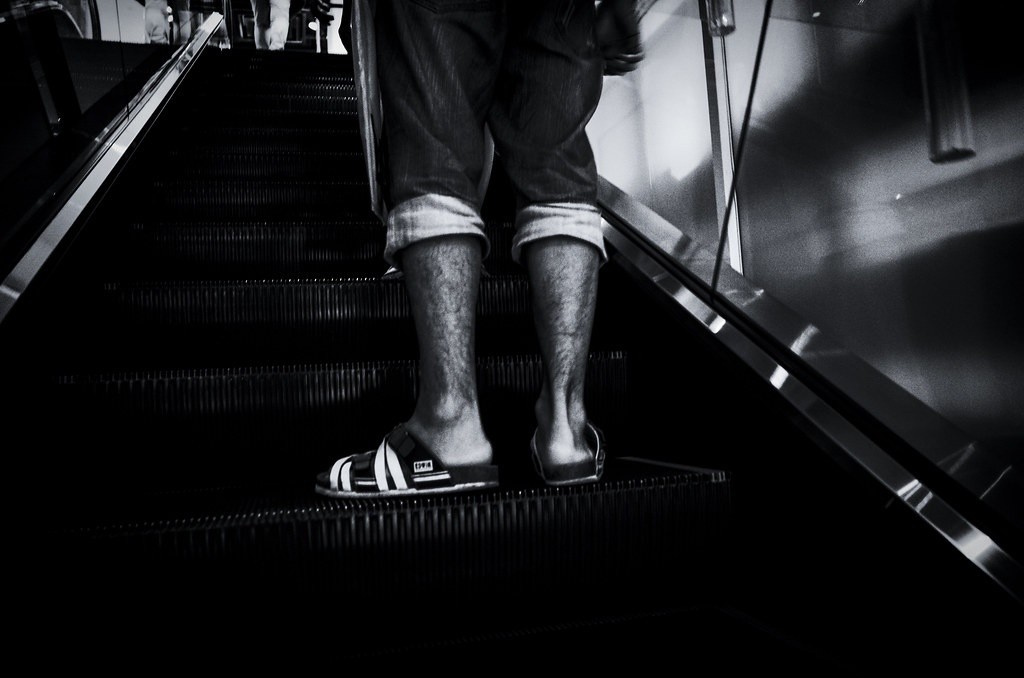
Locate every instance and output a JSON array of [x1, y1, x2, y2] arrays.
[[314, 423, 500, 498], [531, 420, 606, 488]]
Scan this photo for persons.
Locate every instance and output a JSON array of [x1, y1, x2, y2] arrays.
[[318, 0, 607, 500]]
